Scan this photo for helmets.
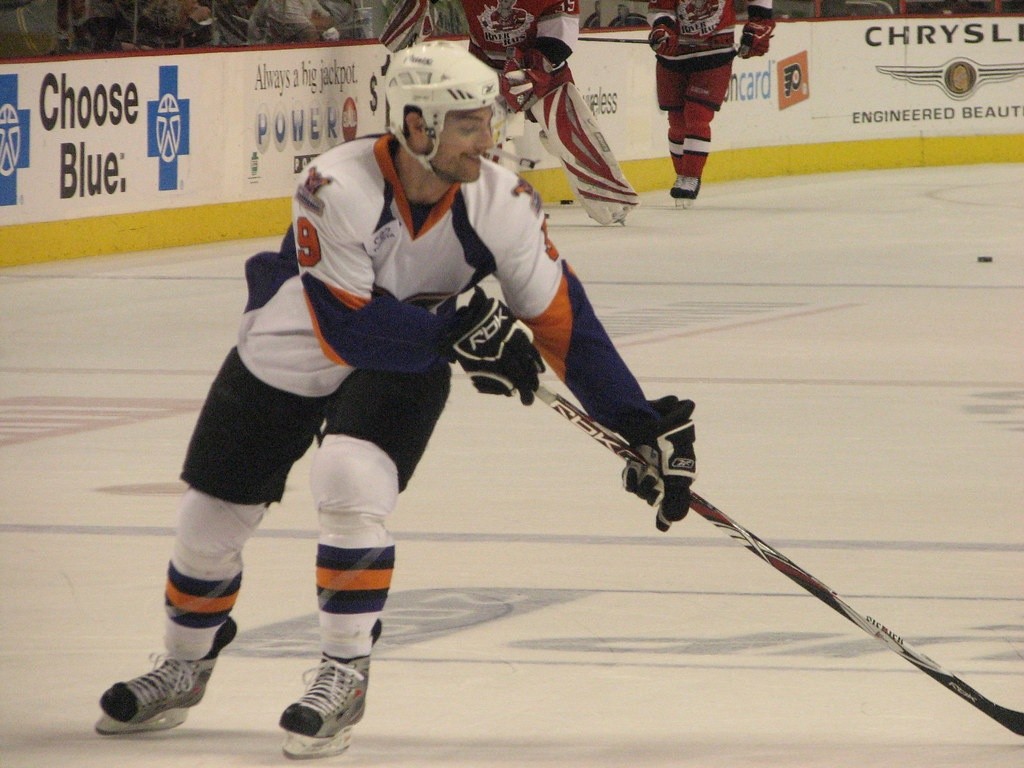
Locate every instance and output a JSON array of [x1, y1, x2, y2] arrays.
[[383, 39, 499, 162]]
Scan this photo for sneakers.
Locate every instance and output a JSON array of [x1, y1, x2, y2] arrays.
[[278, 618, 383, 758], [680, 176, 701, 209], [96, 617, 237, 734], [670, 175, 684, 207]]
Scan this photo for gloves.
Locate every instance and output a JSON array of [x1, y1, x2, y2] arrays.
[[739, 19, 775, 60], [622, 395, 698, 533], [437, 286, 546, 406], [648, 24, 678, 56]]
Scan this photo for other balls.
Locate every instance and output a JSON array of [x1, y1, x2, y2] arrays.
[[977, 256, 993, 262]]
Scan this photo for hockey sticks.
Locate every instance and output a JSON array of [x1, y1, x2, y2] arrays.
[[534, 383, 1024, 737], [575, 37, 741, 49]]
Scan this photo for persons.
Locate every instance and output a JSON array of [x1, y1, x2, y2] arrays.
[[648, 0, 775, 199], [99, 40, 696, 738], [0, 0, 363, 58], [381, 0, 641, 226]]
